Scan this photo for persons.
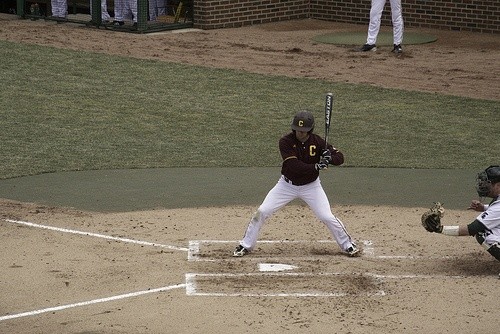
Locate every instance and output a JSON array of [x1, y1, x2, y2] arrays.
[[233, 111, 360, 256], [421, 166, 500, 277], [51, 0, 165, 25], [361, 0, 404, 53]]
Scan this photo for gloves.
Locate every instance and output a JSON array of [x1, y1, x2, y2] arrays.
[[317, 149, 331, 170], [425, 215, 443, 234]]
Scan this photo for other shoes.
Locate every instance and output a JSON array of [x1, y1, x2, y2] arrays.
[[344, 243, 360, 256]]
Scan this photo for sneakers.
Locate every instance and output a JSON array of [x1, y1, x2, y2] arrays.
[[360, 44, 376, 52], [391, 44, 402, 53], [233, 245, 250, 256]]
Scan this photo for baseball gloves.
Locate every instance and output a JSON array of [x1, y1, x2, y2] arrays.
[[421, 200, 444, 233]]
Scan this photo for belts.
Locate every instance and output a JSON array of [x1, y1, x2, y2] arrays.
[[284, 176, 298, 185]]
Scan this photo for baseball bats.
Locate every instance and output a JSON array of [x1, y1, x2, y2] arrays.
[[325, 93, 334, 150]]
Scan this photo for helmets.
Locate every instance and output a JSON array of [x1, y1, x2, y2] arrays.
[[478, 165, 500, 182], [291, 111, 315, 132]]
